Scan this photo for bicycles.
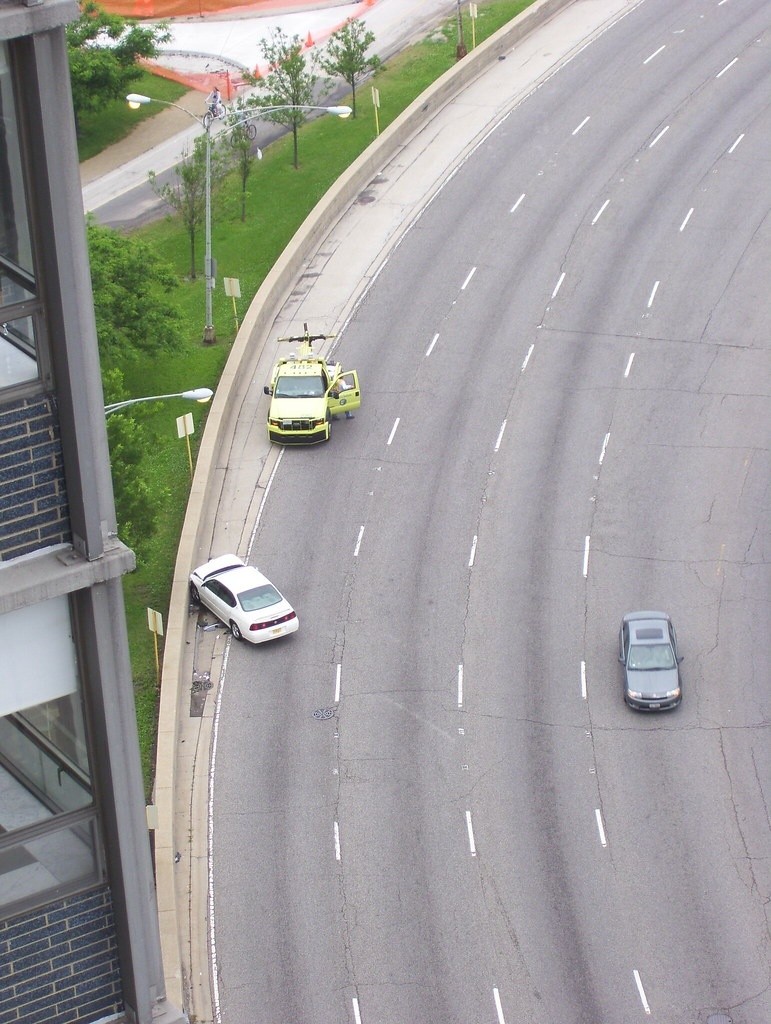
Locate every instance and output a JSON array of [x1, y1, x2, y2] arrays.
[[203, 101, 257, 149]]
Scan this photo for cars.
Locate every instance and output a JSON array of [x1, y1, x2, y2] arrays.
[[264, 323, 360, 445], [618, 611, 684, 711], [190, 554, 300, 644]]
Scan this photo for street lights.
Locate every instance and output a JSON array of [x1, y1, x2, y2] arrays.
[[126, 93, 353, 343]]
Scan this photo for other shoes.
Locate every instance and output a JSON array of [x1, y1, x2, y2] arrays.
[[346, 416, 355, 419], [332, 417, 340, 420]]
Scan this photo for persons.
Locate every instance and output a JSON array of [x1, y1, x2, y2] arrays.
[[331, 373, 355, 419], [205, 86, 222, 114], [238, 110, 249, 138]]
